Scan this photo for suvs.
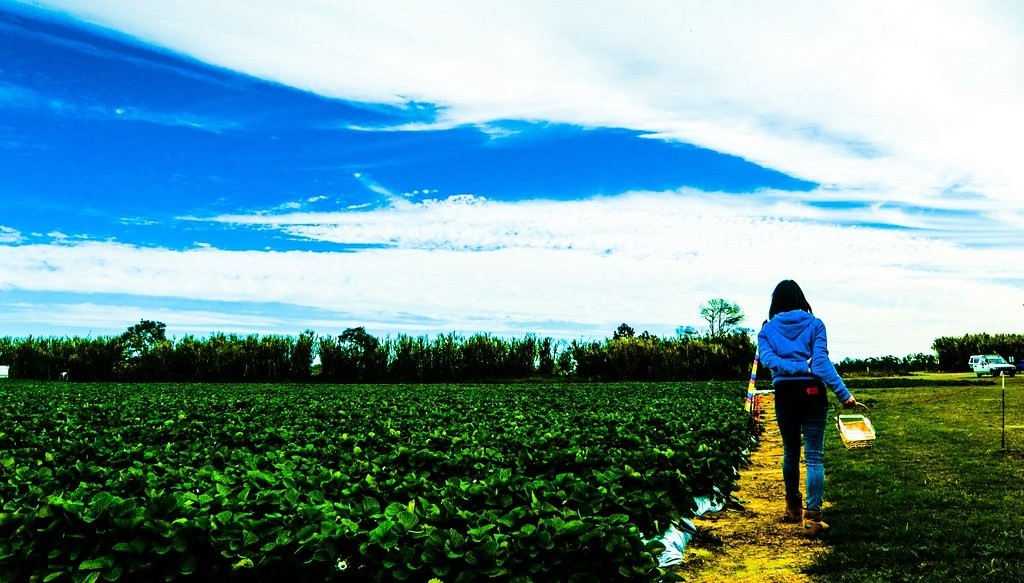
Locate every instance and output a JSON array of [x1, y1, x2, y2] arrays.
[[968, 355, 1016, 378]]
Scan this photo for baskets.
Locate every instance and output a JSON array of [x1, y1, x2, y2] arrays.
[[833, 402, 876, 450]]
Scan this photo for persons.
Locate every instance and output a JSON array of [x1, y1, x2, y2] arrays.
[[757, 279, 859, 536]]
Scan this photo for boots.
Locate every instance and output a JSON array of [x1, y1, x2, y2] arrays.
[[786, 505, 803, 522], [801, 509, 830, 537]]
